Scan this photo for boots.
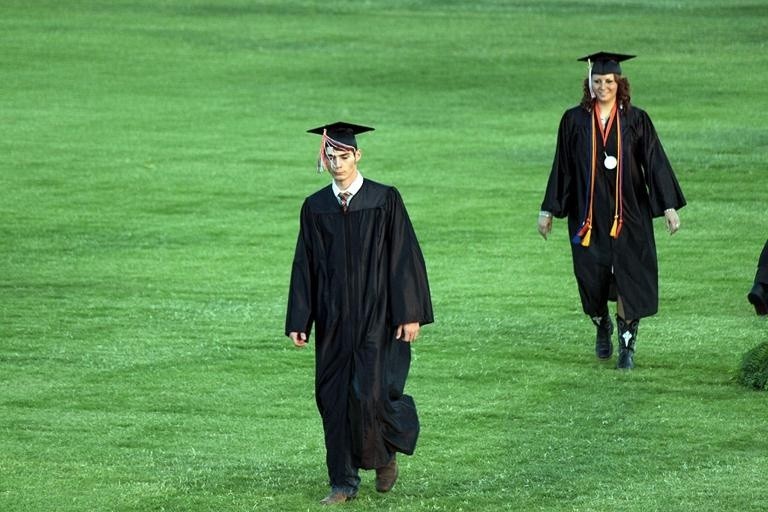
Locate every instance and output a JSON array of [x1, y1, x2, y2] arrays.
[[590, 312, 612, 356], [615, 314, 639, 369]]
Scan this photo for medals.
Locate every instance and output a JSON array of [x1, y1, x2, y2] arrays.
[[604, 156, 617, 169]]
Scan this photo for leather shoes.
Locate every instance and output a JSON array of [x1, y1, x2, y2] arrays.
[[374, 454, 398, 491], [319, 489, 355, 505]]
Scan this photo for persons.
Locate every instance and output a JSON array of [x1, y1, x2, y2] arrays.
[[284, 121, 434, 506], [747, 239, 768, 315], [538, 52, 686, 371]]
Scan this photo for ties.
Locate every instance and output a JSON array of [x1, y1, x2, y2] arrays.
[[338, 193, 352, 212]]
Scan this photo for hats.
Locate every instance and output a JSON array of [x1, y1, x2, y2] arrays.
[[307, 122, 375, 174], [578, 53, 636, 98]]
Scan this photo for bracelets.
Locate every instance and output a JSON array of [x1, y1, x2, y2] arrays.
[[539, 211, 552, 217]]
